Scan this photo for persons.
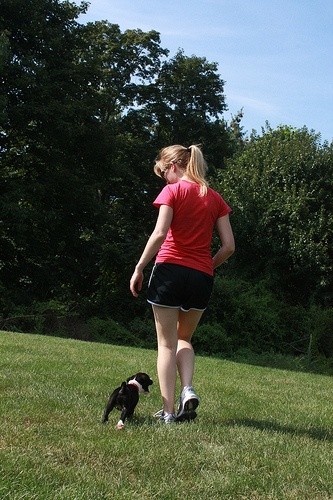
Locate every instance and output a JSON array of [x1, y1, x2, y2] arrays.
[[129, 143, 236, 425]]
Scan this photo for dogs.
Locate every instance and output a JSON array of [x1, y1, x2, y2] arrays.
[[101, 373, 153, 429]]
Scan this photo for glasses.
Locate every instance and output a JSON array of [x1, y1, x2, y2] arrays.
[[160, 164, 170, 179]]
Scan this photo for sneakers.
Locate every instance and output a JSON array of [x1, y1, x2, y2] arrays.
[[151, 409, 175, 424], [175, 386, 199, 422]]
